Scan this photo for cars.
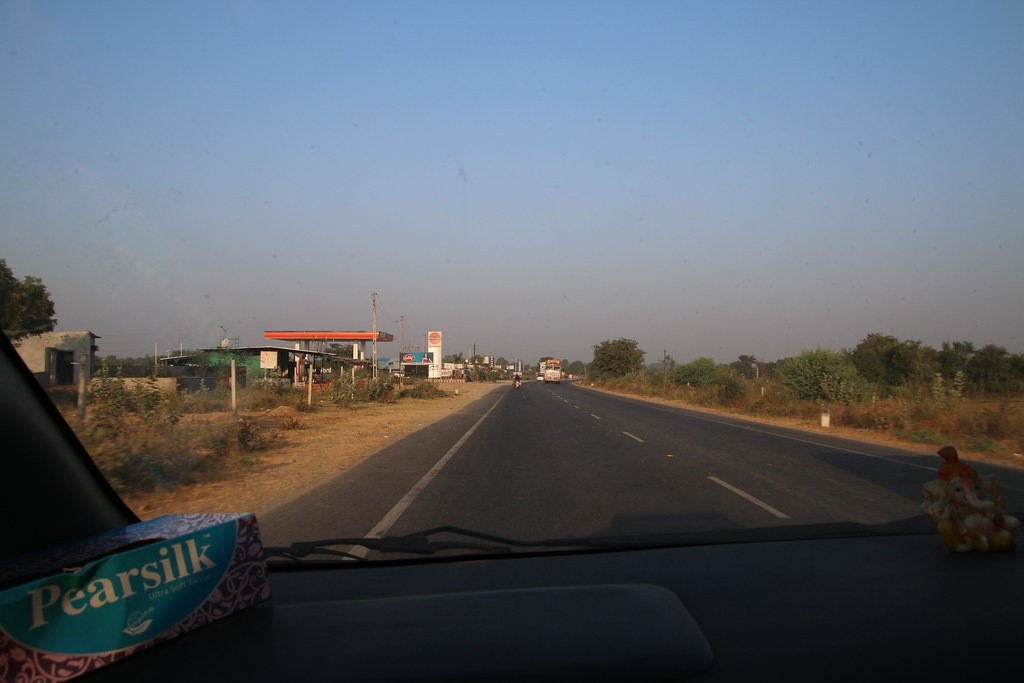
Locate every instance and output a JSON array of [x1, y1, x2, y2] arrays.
[[536, 374, 544, 381]]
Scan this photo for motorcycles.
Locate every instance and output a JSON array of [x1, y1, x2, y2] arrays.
[[512, 379, 522, 389]]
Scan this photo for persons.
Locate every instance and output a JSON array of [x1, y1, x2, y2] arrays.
[[514, 373, 522, 385], [421, 353, 433, 363]]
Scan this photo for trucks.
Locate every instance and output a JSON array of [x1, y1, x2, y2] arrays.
[[541, 364, 562, 383]]
